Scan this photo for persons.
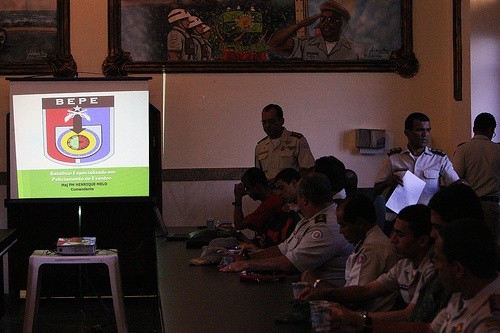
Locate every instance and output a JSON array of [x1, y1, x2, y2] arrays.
[[219, 103, 500, 333]]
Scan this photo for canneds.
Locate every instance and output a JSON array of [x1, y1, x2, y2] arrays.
[[207, 217, 214, 229]]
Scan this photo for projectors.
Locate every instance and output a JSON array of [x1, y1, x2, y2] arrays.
[[57, 236, 96, 255]]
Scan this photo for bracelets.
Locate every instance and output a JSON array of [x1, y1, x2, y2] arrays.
[[232, 202, 242, 205], [313, 278, 324, 288], [360, 310, 367, 325]]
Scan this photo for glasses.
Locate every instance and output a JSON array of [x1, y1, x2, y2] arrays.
[[260, 119, 276, 125]]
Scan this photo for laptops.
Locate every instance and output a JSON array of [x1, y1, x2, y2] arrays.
[[153, 206, 189, 241]]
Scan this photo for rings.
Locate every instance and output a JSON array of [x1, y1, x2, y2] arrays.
[[229, 266, 231, 270]]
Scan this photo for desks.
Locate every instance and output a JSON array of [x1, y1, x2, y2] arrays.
[[156, 225, 346, 333], [23, 249, 126, 333]]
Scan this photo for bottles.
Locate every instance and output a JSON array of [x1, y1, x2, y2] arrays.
[[221, 221, 232, 232]]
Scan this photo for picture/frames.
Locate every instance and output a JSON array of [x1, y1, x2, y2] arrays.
[[102, 0, 419, 78], [0, 0, 77, 77]]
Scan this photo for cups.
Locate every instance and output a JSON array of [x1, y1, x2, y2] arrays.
[[309, 300, 328, 329], [312, 304, 331, 332], [224, 257, 234, 268], [292, 282, 308, 300]]
